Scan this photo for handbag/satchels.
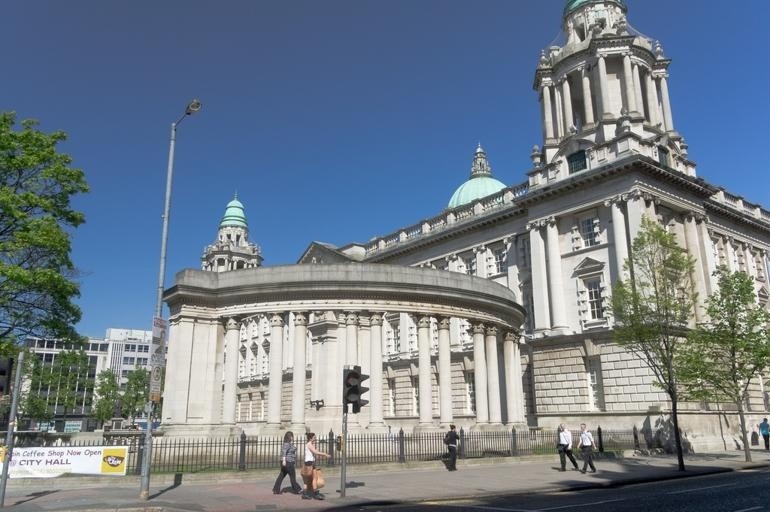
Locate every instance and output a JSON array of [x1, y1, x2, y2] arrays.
[[301, 465, 313, 477], [317, 469, 325, 489], [444, 431, 450, 445]]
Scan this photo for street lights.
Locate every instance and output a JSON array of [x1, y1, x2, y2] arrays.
[[139, 94, 204, 500]]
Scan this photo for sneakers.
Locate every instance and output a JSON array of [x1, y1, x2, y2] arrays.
[[303, 493, 312, 499], [314, 494, 325, 500]]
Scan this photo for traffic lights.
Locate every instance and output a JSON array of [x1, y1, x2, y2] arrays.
[[341, 369, 361, 404], [351, 365, 371, 412]]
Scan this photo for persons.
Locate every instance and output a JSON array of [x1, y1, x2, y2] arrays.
[[446, 424, 460, 471], [556, 422, 580, 471], [575, 422, 598, 474], [272, 430, 303, 494], [300, 432, 332, 499], [758, 418, 770, 450]]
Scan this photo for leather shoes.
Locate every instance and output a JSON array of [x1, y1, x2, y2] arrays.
[[559, 468, 595, 473]]
